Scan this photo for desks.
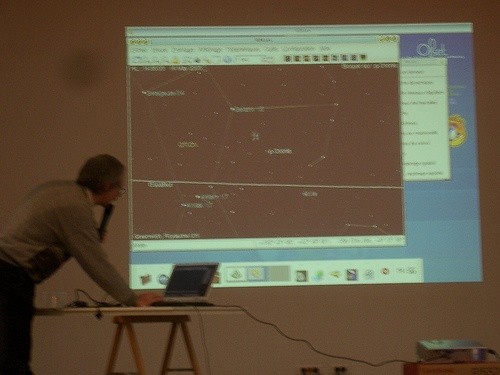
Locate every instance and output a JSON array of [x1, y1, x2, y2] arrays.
[[34, 301, 243, 375]]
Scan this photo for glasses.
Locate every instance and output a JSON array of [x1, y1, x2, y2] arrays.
[[118, 184, 126, 194]]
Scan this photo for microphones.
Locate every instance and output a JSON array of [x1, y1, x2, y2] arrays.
[[98, 204, 115, 240]]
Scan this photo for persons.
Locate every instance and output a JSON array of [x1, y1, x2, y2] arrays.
[[1, 154, 166, 375]]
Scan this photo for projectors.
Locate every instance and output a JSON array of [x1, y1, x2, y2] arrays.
[[416, 338, 490, 361]]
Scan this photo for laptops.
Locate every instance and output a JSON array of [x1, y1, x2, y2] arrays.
[[161, 263, 219, 302]]
